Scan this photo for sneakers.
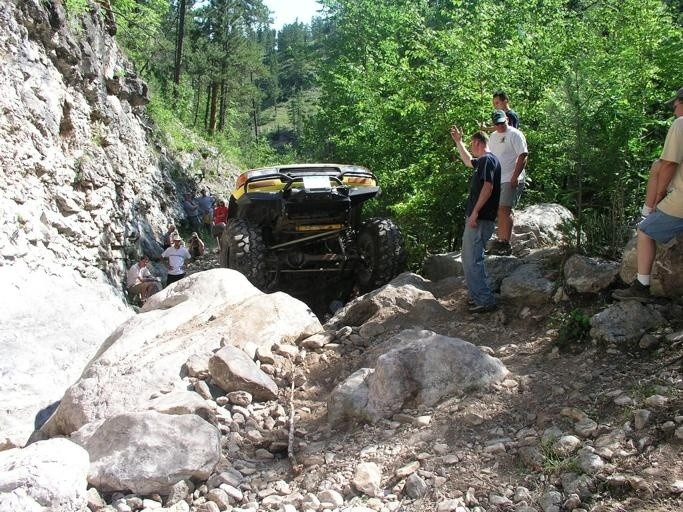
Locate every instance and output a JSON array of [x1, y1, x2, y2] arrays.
[[629, 215, 646, 229], [484, 239, 512, 256], [468, 299, 496, 313], [611, 279, 652, 303]]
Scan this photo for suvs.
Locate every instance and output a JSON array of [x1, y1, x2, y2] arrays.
[[218, 163, 409, 317]]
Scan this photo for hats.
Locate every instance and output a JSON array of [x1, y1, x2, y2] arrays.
[[664, 87, 683, 105], [172, 234, 183, 241], [490, 109, 506, 123]]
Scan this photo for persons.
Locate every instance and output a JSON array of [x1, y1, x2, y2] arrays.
[[125, 188, 229, 308], [492, 93, 520, 129], [449, 124, 501, 313], [611, 88, 682, 304], [483, 110, 528, 257], [627, 159, 664, 230]]
